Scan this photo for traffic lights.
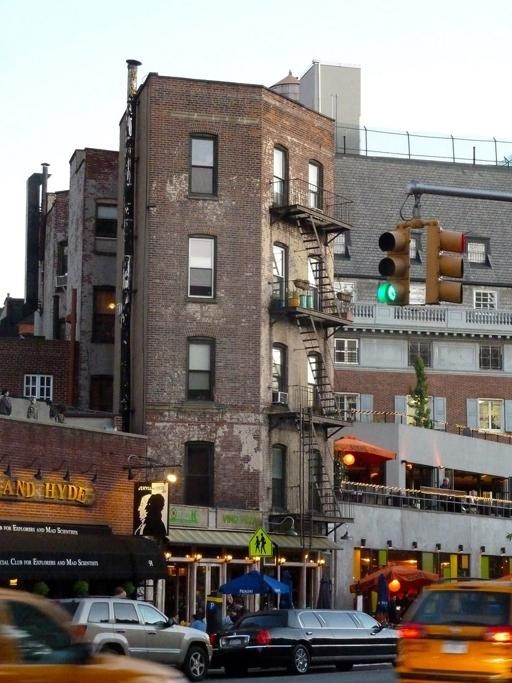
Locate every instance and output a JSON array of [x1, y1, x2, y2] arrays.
[[424, 221, 466, 306], [376, 226, 410, 306]]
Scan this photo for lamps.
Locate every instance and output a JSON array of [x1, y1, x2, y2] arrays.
[[25, 456, 43, 481], [52, 459, 71, 482], [126, 453, 142, 480], [81, 463, 100, 484], [266, 516, 298, 537], [327, 523, 353, 541], [1, 453, 13, 479]]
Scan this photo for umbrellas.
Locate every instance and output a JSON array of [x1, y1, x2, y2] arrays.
[[218, 570, 290, 614]]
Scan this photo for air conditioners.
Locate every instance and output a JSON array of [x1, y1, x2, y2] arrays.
[[272, 391, 289, 405]]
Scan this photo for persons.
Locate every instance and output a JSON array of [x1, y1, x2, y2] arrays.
[[440, 477, 451, 489], [113, 586, 127, 597], [381, 582, 415, 621], [0, 390, 12, 415], [27, 398, 39, 419], [168, 587, 253, 641]]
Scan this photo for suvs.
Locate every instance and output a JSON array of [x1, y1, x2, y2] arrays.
[[46, 598, 216, 681], [392, 577, 512, 683]]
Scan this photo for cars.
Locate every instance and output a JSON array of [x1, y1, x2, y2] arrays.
[[0, 588, 190, 682], [210, 607, 402, 678]]
